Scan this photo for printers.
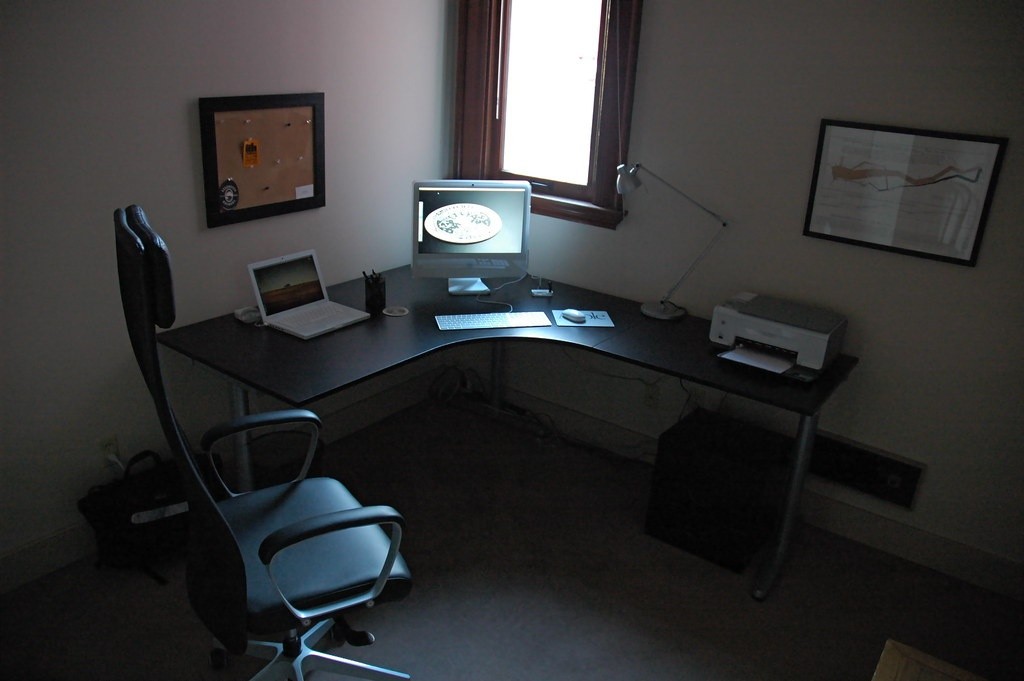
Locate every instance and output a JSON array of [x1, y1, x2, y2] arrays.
[[710, 291, 848, 383]]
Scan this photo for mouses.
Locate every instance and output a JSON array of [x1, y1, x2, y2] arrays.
[[562, 309, 586, 322]]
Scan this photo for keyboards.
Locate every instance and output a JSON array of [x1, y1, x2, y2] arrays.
[[435, 312, 552, 330]]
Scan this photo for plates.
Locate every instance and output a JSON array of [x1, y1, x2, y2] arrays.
[[425, 204, 502, 243]]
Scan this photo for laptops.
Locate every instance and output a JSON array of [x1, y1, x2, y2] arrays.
[[248, 249, 370, 339]]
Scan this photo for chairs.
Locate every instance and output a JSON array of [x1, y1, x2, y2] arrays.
[[113, 204, 417, 681]]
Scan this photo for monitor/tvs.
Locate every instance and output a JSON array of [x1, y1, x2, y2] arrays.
[[412, 180, 532, 295]]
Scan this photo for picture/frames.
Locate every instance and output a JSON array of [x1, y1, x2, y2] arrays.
[[803, 119, 1011, 268], [198, 92, 326, 227]]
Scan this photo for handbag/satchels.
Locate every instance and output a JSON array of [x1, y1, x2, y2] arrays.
[[77, 451, 223, 571]]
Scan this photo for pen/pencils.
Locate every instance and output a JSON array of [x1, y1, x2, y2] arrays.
[[362, 268, 383, 281]]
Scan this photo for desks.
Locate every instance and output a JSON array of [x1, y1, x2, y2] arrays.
[[158, 265, 859, 604]]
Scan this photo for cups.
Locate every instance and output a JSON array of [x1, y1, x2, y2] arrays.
[[365, 277, 385, 310]]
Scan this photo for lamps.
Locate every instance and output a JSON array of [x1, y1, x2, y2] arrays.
[[617, 162, 729, 320]]
